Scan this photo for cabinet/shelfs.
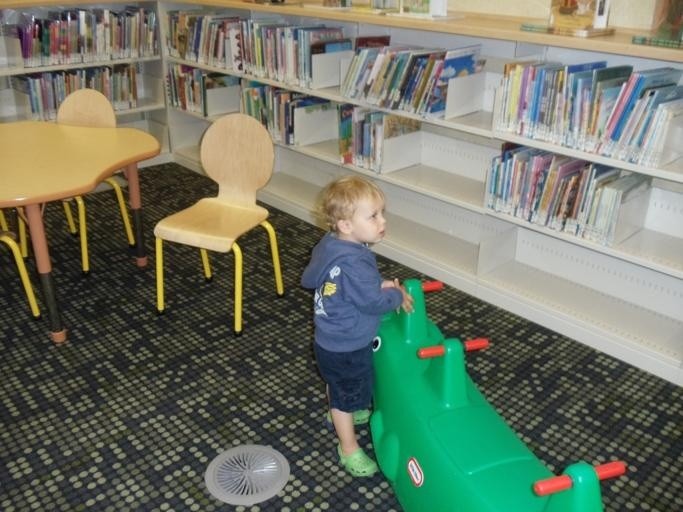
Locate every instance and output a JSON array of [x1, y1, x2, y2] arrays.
[[0, 0, 171, 177], [171, 0, 683, 391]]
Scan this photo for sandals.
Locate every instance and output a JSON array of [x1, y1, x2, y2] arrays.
[[338, 440, 378, 478], [326, 407, 370, 426]]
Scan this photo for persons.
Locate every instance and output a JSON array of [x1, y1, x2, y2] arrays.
[[300, 173, 416, 478]]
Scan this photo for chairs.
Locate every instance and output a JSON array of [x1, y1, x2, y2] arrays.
[[151, 112, 288, 333], [0, 84, 134, 316]]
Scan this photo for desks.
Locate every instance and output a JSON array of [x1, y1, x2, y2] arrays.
[[0, 121, 162, 345]]
